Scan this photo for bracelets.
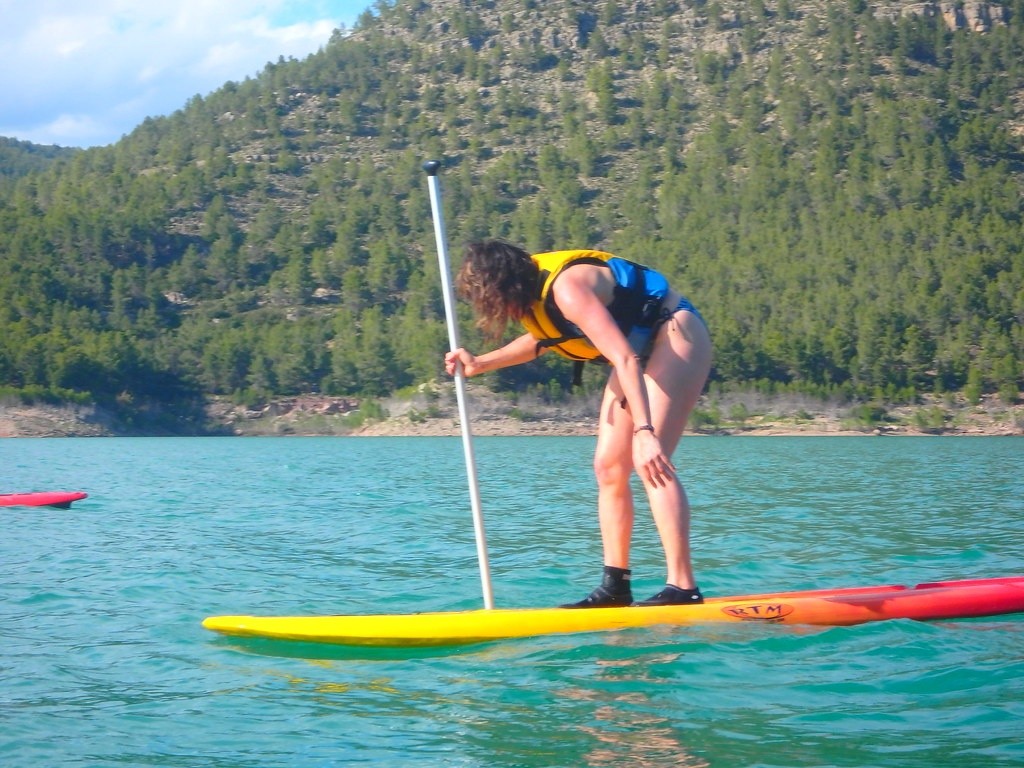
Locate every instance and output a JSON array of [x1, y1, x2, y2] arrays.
[[635, 425, 655, 432]]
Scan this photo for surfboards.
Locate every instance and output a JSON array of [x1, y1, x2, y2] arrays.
[[202, 574, 1024, 642], [0, 492, 88, 506]]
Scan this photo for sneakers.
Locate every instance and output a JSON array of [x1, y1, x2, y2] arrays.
[[628, 584, 704, 606], [559, 583, 635, 609]]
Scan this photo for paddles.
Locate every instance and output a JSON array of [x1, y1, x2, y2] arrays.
[[421, 160, 498, 610]]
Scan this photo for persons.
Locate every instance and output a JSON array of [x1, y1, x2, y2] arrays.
[[445, 239, 711, 609]]
